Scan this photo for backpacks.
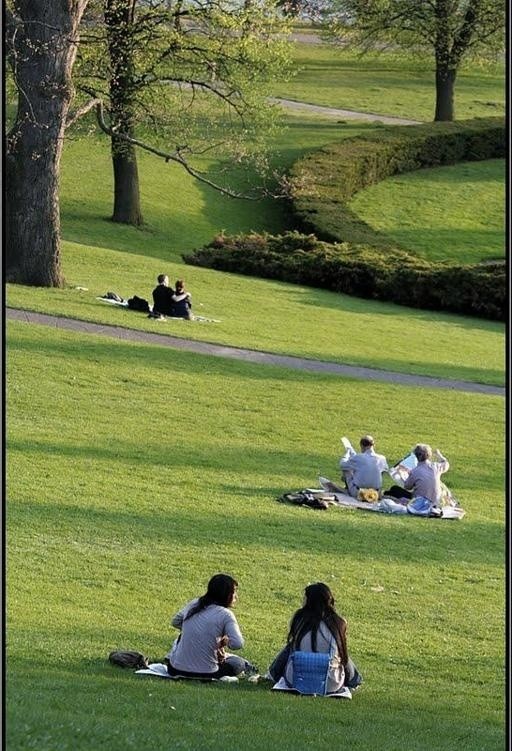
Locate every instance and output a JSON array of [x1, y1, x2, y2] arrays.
[[290, 633, 333, 697]]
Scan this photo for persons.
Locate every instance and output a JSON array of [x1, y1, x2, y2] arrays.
[[382, 441, 450, 509], [261, 581, 360, 696], [172, 280, 193, 320], [153, 273, 192, 317], [339, 434, 389, 502], [164, 573, 259, 680]]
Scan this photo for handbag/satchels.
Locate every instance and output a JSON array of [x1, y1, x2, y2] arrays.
[[127, 295, 149, 312], [107, 291, 122, 302], [110, 651, 144, 668]]
